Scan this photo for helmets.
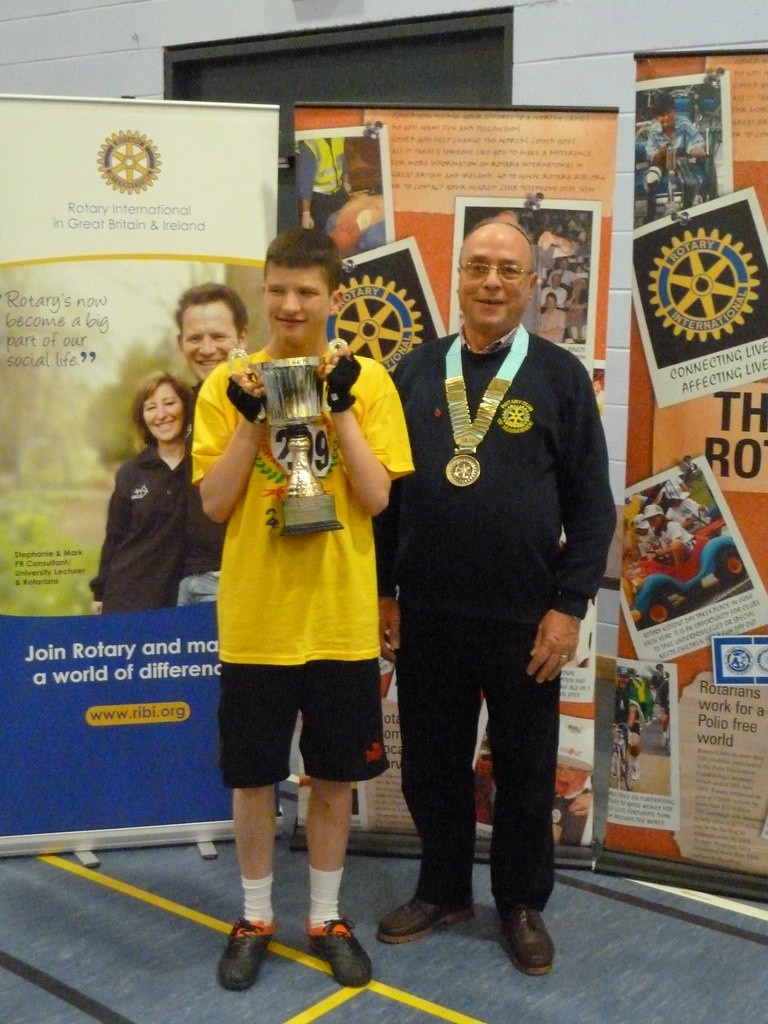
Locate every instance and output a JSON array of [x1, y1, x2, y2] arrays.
[[618, 667, 628, 676]]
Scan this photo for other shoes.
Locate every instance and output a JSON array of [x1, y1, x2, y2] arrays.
[[630, 758, 640, 780]]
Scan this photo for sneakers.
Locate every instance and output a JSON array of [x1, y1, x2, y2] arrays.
[[307, 916, 372, 987], [219, 918, 276, 990]]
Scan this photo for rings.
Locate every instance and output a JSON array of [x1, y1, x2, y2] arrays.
[[560, 655, 566, 658]]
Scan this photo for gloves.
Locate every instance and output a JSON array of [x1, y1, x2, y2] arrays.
[[302, 211, 314, 229]]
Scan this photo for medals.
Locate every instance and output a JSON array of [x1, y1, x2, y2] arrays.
[[446, 455, 481, 487]]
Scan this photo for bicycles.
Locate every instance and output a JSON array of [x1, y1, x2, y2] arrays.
[[611, 723, 632, 791]]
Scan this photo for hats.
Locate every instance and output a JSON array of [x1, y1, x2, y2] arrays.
[[478, 735, 492, 754], [556, 724, 593, 770], [665, 485, 690, 500], [628, 494, 648, 504], [634, 514, 650, 530], [641, 504, 664, 522]]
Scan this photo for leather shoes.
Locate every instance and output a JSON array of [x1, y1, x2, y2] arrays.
[[376, 894, 474, 944], [503, 904, 554, 974]]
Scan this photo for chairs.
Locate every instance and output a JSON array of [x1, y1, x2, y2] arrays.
[[635, 122, 710, 225]]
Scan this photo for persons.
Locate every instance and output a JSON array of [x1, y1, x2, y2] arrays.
[[496, 210, 593, 345], [550, 746, 593, 845], [630, 476, 708, 557], [648, 664, 669, 738], [190, 229, 414, 989], [88, 371, 195, 612], [173, 282, 248, 606], [613, 666, 642, 780], [643, 93, 705, 224], [473, 737, 496, 827], [371, 219, 618, 972], [297, 137, 352, 233]]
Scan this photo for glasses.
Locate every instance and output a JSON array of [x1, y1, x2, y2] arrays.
[[460, 263, 533, 279], [480, 755, 493, 761]]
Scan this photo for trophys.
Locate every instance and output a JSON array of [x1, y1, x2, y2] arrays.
[[227, 338, 349, 537]]
